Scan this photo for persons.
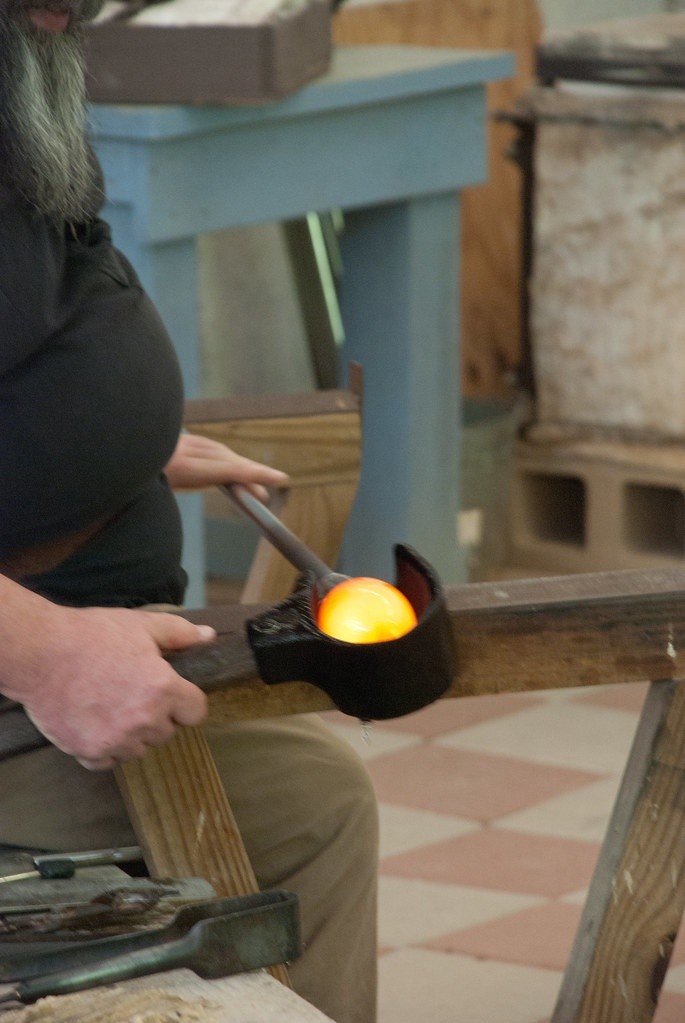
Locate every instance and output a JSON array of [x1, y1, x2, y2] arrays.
[[0, 0, 379, 1023]]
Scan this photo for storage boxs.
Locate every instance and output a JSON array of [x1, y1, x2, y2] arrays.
[[83, 0, 334, 108]]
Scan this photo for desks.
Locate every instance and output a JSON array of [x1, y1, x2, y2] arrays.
[[89, 47, 519, 608]]
